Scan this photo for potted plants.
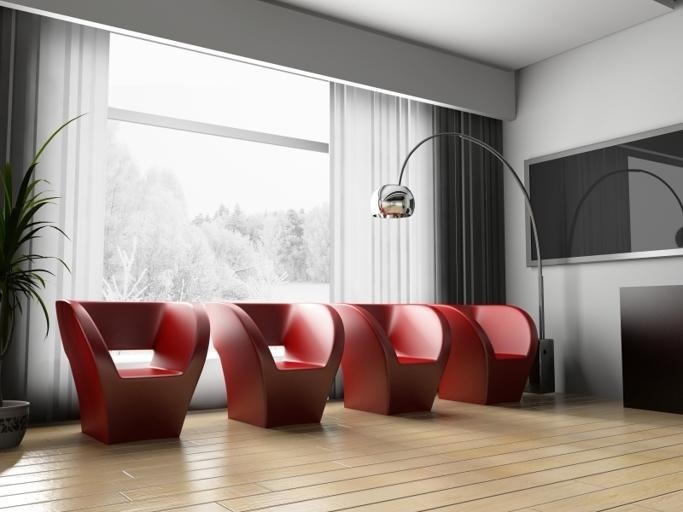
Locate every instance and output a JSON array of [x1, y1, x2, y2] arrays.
[[1, 113, 89, 450]]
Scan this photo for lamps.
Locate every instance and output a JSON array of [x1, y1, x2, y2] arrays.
[[373, 128, 559, 396]]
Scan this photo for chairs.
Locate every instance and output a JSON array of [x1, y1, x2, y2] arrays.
[[334, 302, 451, 416], [208, 300, 346, 429], [433, 305, 540, 402], [55, 300, 210, 444]]
